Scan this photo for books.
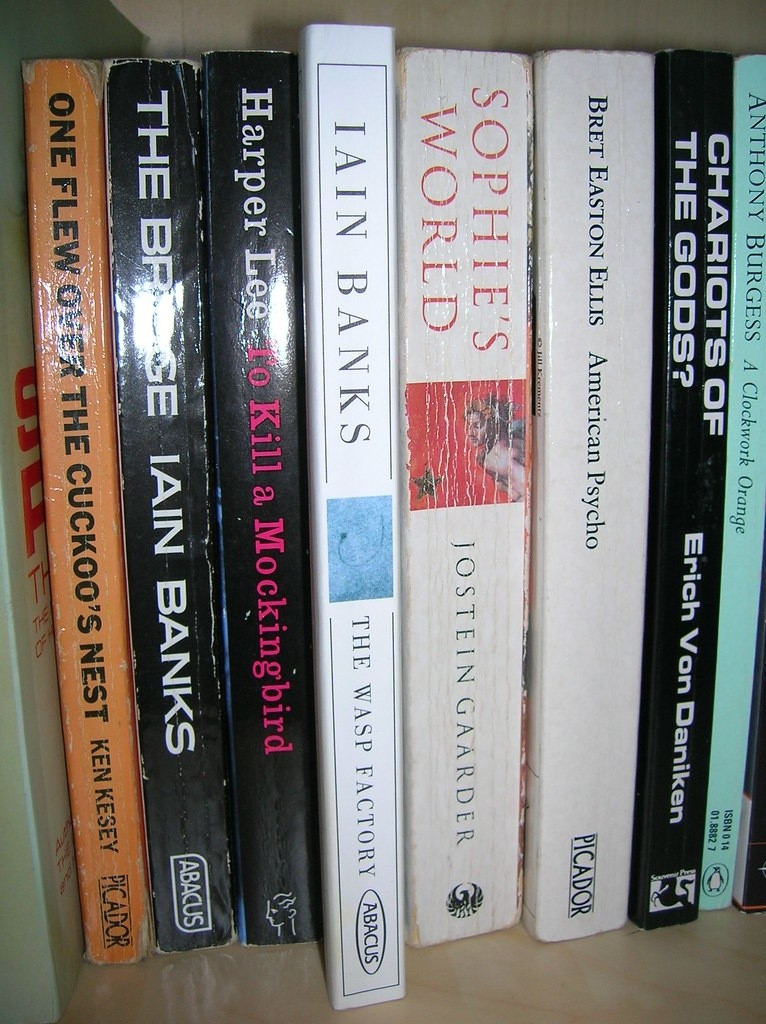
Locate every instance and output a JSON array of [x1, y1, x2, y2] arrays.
[[630, 41, 765, 934], [521, 52, 655, 943], [292, 18, 406, 1010], [99, 53, 239, 954], [2, 0, 151, 1024], [394, 44, 532, 947], [202, 48, 318, 941]]
